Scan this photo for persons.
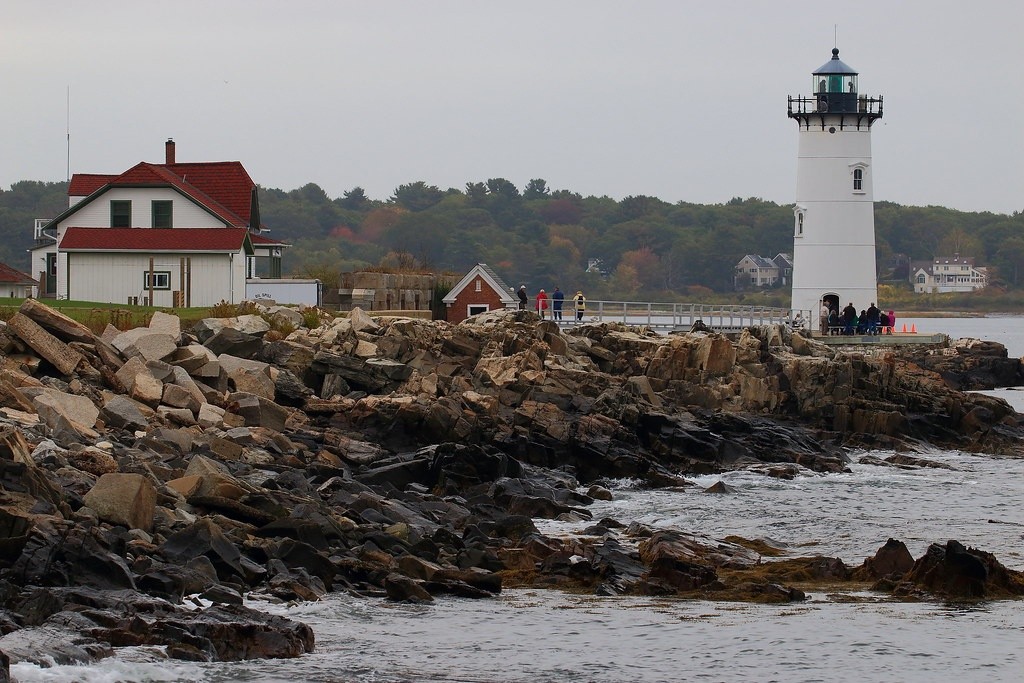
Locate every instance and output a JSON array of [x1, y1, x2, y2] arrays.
[[518, 285, 527, 309], [820, 300, 895, 336], [573, 291, 586, 321], [536, 289, 548, 319], [819, 80, 826, 92], [552, 287, 564, 320], [848, 82, 855, 93]]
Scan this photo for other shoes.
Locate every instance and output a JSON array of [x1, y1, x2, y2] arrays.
[[554, 319, 557, 321], [559, 319, 562, 321]]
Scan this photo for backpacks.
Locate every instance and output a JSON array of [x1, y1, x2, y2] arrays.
[[578, 296, 583, 305]]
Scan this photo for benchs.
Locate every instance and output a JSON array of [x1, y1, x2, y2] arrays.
[[876, 326, 891, 334], [828, 326, 857, 336]]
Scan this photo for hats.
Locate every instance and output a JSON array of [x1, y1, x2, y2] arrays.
[[520, 285, 526, 289], [796, 314, 801, 317], [880, 311, 885, 314], [831, 310, 836, 314], [510, 287, 514, 292], [578, 290, 582, 294], [871, 302, 875, 305], [824, 301, 830, 306], [554, 286, 559, 289]]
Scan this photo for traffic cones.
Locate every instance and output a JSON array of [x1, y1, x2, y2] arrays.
[[891, 326, 895, 332], [883, 327, 887, 332], [902, 323, 905, 330], [911, 323, 916, 332]]
